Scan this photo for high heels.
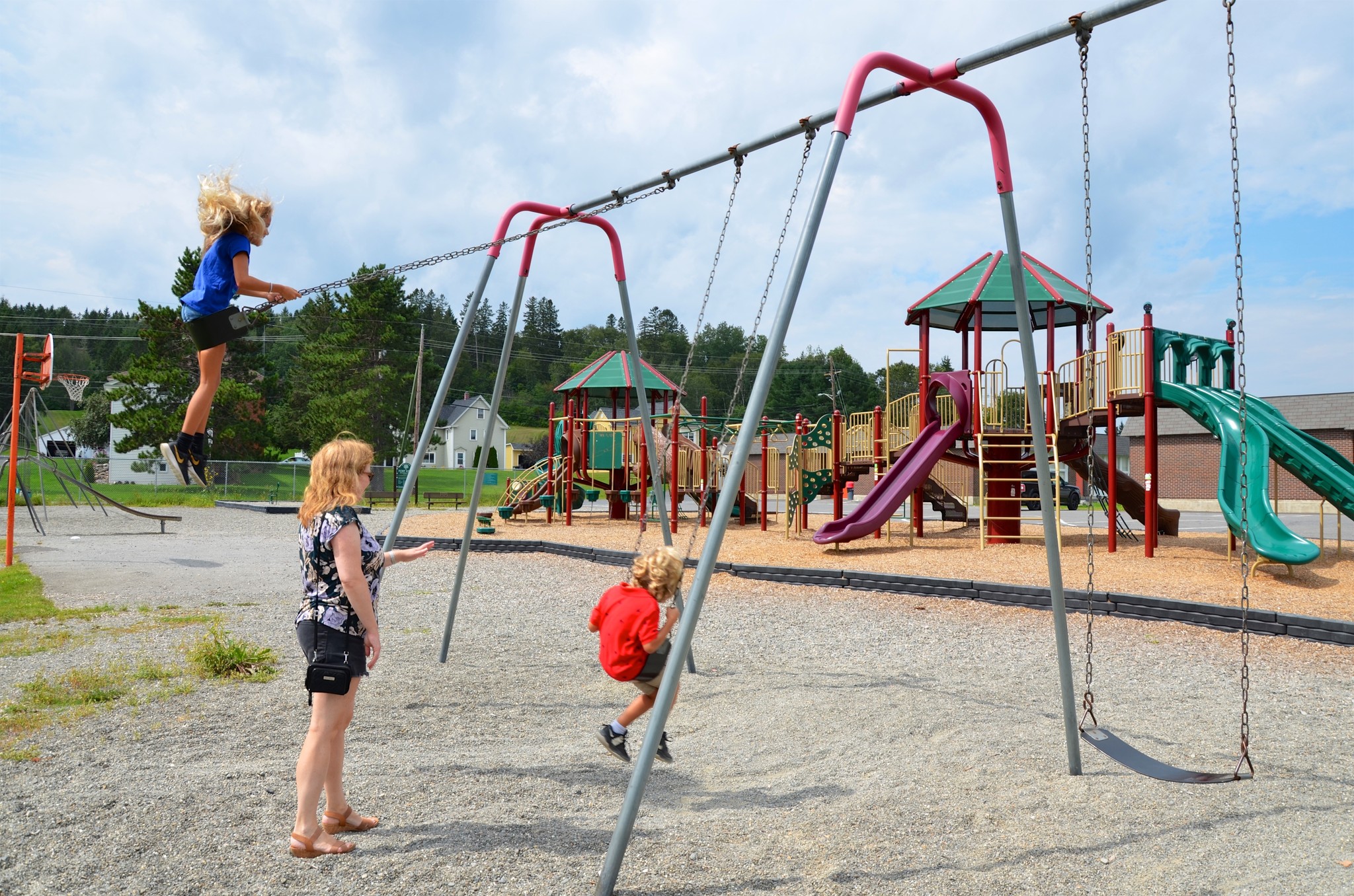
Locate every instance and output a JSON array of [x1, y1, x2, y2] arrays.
[[290, 827, 356, 858], [321, 805, 379, 835]]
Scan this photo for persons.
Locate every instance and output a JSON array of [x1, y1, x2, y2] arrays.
[[283, 431, 436, 858], [160, 164, 301, 488], [587, 544, 684, 764]]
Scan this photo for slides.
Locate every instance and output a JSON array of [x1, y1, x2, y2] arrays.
[[677, 432, 758, 518], [813, 422, 964, 547], [1057, 436, 1181, 537], [26, 454, 182, 522], [507, 460, 577, 515], [1221, 385, 1353, 523], [1164, 375, 1324, 570]]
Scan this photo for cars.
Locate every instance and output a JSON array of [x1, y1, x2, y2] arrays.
[[276, 456, 312, 466]]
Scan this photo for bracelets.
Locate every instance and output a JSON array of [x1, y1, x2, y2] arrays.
[[389, 550, 396, 565], [268, 282, 272, 293]]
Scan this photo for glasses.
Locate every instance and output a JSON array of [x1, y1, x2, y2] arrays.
[[362, 471, 374, 481]]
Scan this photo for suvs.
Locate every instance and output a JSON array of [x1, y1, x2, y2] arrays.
[[1019, 470, 1081, 511]]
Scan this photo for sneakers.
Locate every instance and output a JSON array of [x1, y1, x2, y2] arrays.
[[188, 449, 209, 488], [160, 441, 191, 486], [639, 731, 674, 763], [596, 723, 630, 762]]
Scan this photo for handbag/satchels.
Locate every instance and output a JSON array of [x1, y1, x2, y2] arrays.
[[304, 662, 352, 705]]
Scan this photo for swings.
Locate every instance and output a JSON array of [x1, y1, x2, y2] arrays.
[[1072, 0, 1257, 785], [188, 186, 674, 355], [629, 125, 816, 683]]
